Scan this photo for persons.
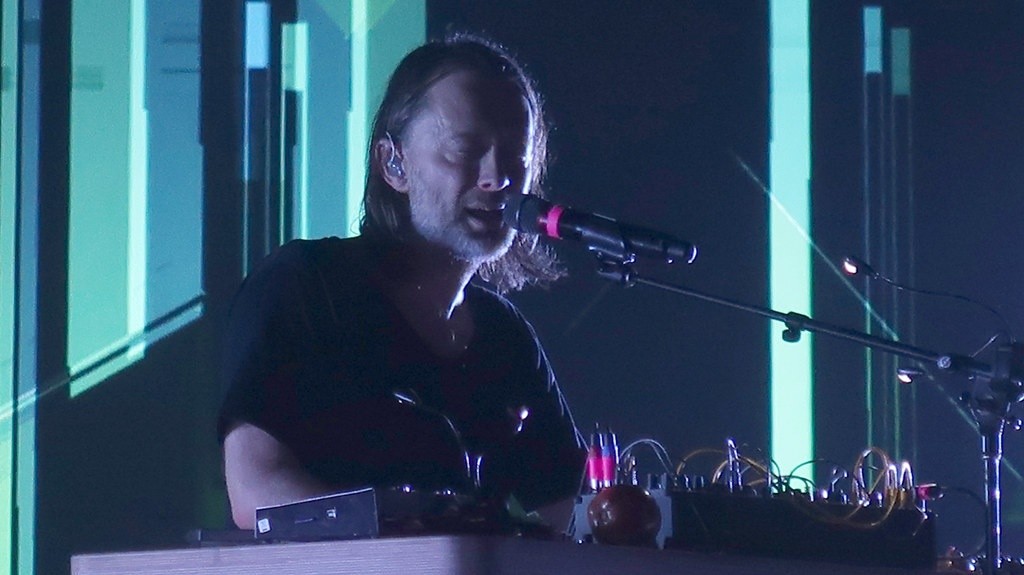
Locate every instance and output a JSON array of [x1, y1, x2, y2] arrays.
[[215, 39, 589, 540]]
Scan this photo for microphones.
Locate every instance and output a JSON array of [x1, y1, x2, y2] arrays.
[[501, 192, 697, 265]]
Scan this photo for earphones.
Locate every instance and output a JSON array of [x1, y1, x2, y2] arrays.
[[386, 159, 405, 177]]
